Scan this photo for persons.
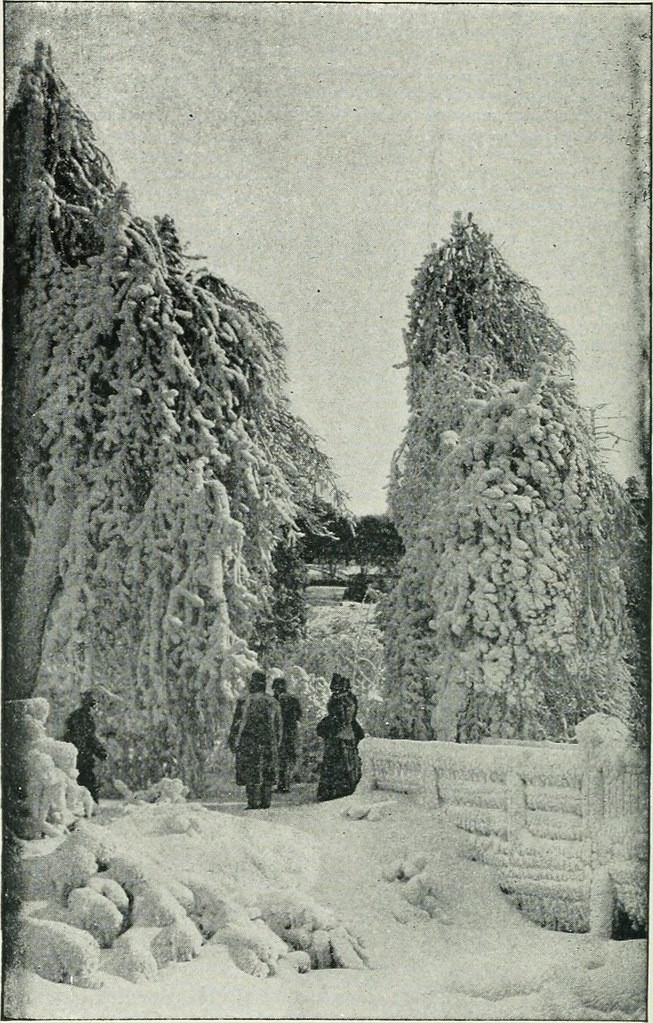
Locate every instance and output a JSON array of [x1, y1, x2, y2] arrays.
[[62, 689, 107, 804], [229, 672, 282, 810], [271, 678, 302, 793], [317, 674, 364, 802]]
